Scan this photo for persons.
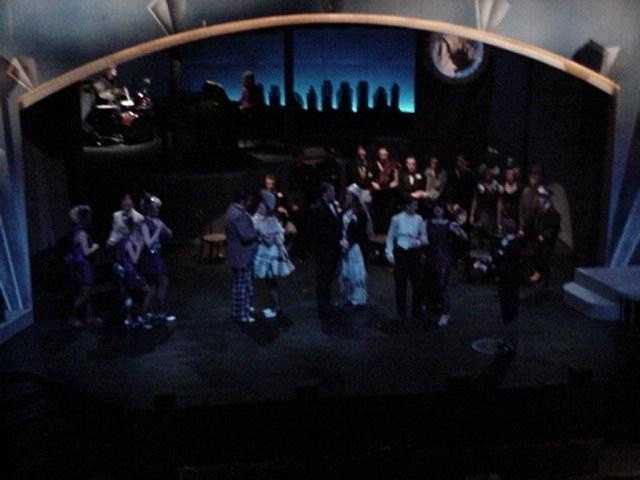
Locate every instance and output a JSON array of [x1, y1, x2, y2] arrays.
[[232, 67, 262, 149], [90, 62, 127, 145], [60, 145, 564, 359]]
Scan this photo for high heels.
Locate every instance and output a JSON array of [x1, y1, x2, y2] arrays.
[[124, 310, 176, 331]]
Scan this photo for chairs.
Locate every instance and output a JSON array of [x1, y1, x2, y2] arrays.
[[195, 208, 229, 264]]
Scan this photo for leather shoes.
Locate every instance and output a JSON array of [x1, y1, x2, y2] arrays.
[[241, 307, 256, 323]]
